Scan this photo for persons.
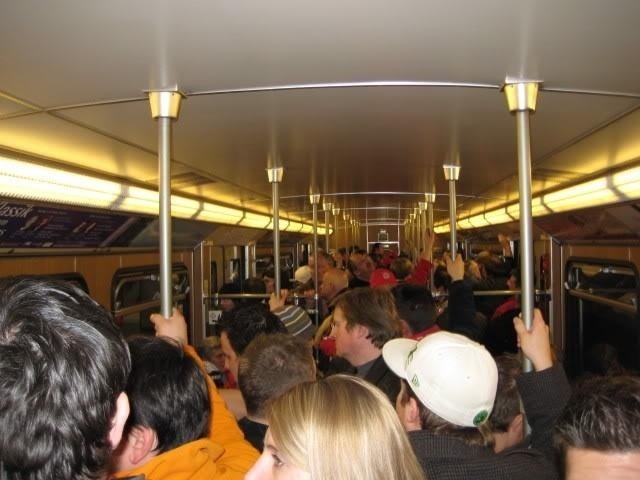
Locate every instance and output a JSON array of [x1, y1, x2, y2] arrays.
[[0, 274, 144, 480], [111, 309, 261, 480], [556, 371, 640, 480], [245, 374, 427, 480], [217, 244, 527, 452], [383, 308, 571, 480]]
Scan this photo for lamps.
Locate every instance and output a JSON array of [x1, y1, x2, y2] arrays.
[[1, 144, 334, 236], [433, 157, 639, 236]]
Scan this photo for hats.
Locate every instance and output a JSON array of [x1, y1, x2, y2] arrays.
[[370, 269, 397, 288], [382, 331, 498, 428], [294, 266, 313, 283]]
